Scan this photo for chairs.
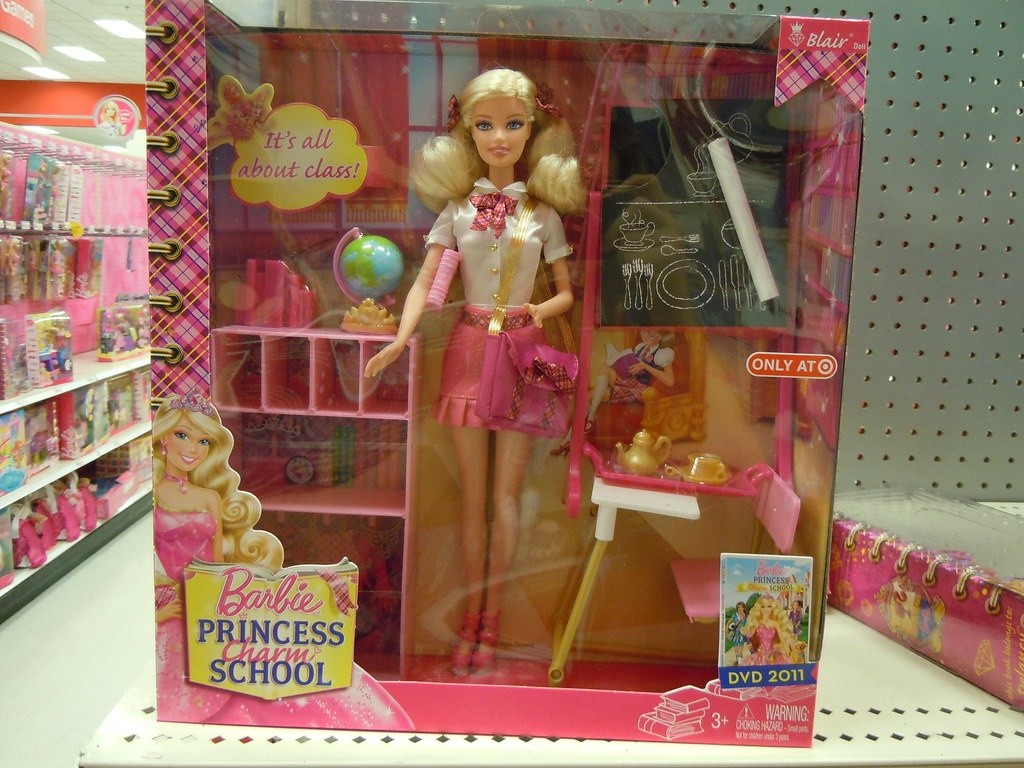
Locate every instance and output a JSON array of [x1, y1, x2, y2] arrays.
[[669, 465, 801, 623]]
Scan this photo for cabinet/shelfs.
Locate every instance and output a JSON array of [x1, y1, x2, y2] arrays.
[[0, 121, 154, 621], [210, 326, 425, 679]]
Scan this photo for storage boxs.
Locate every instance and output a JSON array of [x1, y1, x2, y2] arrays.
[[828, 482, 1024, 708], [355, 588, 400, 656], [144, 0, 871, 749], [0, 300, 153, 590]]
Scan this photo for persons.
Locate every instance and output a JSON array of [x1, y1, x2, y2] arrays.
[[2, 235, 67, 300], [0, 156, 63, 219], [366, 68, 586, 678]]
